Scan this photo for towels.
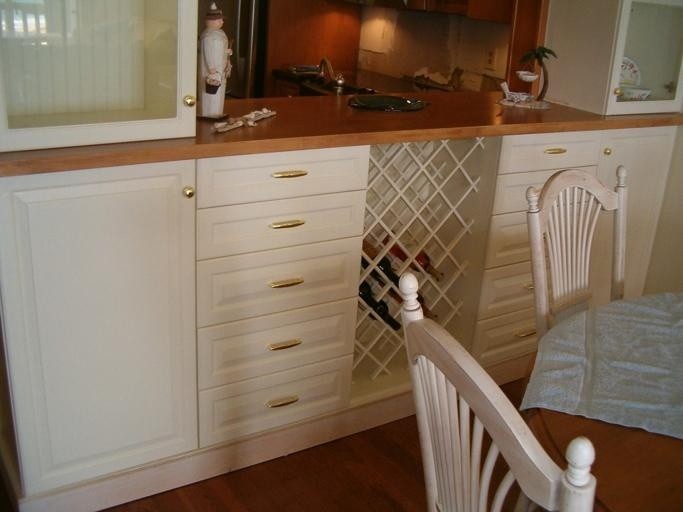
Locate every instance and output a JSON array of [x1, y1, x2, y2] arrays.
[[520, 291, 683, 439]]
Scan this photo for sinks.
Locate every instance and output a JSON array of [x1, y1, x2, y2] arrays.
[[304, 81, 379, 96], [351, 95, 427, 112]]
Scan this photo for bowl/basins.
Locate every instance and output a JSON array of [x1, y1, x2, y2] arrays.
[[617, 85, 652, 102]]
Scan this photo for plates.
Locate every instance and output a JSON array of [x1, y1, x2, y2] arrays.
[[619, 56, 640, 89], [348, 93, 426, 113]]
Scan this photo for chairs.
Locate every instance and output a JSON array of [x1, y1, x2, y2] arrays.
[[398, 272, 597, 511], [525, 163, 629, 348]]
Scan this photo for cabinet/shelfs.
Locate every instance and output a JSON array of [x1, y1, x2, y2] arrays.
[[0, 158, 198, 497], [1, 1, 198, 149], [469, 131, 601, 368], [587, 125, 676, 303], [195, 143, 370, 457], [538, 1, 683, 118]]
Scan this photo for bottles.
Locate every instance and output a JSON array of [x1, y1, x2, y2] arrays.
[[357, 241, 445, 331]]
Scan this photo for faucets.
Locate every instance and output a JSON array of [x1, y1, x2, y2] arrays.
[[397, 96, 423, 109], [318, 58, 346, 96]]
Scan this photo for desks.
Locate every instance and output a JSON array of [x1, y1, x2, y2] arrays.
[[520, 295, 683, 512]]
